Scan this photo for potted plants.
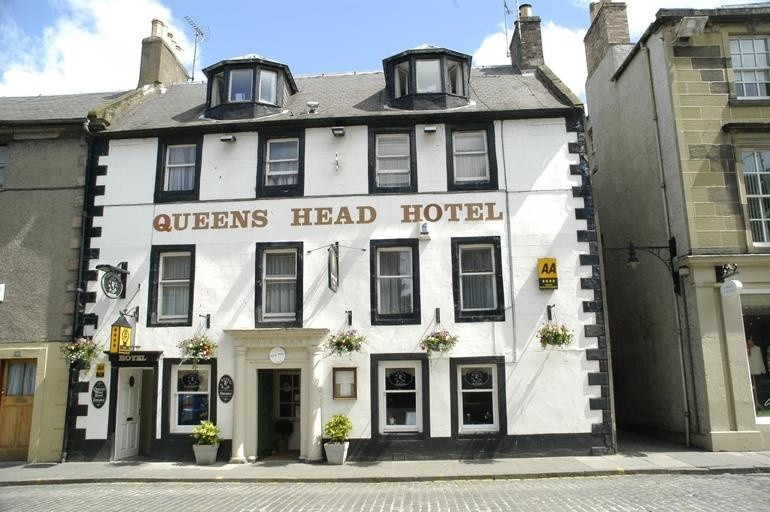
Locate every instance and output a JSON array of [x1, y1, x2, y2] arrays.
[[187, 419, 225, 465], [274, 419, 294, 451], [324, 413, 353, 465]]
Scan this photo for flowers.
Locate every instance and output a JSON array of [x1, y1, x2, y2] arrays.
[[322, 328, 367, 361], [536, 320, 577, 351], [59, 336, 104, 365], [418, 328, 459, 361], [175, 335, 218, 371]]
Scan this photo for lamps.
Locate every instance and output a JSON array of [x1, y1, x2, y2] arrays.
[[331, 126, 346, 137], [673, 14, 710, 43], [625, 241, 641, 271], [110, 309, 133, 356], [220, 135, 236, 142], [422, 126, 437, 133]]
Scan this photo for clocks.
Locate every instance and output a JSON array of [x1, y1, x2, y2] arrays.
[[388, 368, 413, 387], [464, 368, 490, 386]]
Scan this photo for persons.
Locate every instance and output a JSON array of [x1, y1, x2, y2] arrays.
[[746, 334, 767, 405]]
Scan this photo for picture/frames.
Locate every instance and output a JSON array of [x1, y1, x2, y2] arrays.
[[332, 366, 357, 400]]
